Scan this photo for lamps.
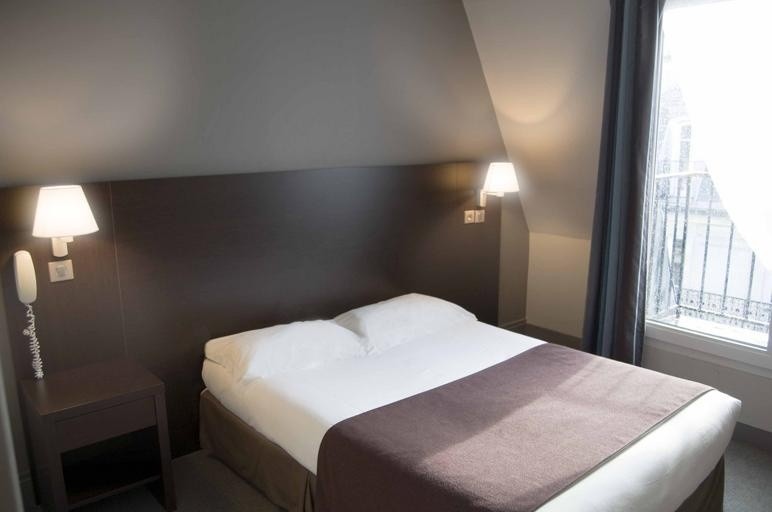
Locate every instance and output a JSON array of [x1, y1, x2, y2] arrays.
[[479, 160, 520, 208], [31, 183, 100, 258]]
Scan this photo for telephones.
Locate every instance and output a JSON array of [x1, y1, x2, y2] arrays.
[[13, 250, 37, 304]]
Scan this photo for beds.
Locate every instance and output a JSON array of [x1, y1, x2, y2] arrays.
[[189, 291, 742, 512]]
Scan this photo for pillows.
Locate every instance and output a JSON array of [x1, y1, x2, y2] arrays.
[[203, 319, 366, 383], [332, 293, 478, 356]]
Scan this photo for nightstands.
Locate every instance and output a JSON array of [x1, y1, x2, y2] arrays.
[[20, 358, 177, 511]]
